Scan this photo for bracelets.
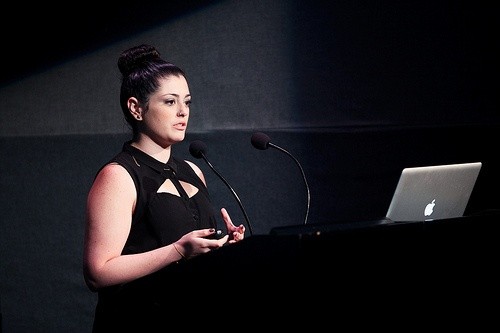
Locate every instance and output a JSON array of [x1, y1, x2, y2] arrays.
[[173, 243, 185, 257]]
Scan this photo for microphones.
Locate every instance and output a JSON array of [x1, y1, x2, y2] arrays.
[[188, 141, 254, 237], [251, 133, 311, 225]]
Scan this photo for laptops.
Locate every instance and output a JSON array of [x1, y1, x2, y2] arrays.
[[385, 161, 483, 221]]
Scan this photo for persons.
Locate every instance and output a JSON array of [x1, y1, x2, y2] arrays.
[[83, 45, 245, 333]]
